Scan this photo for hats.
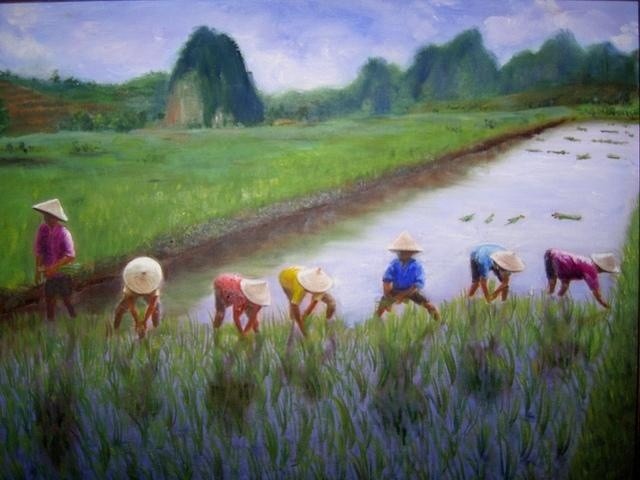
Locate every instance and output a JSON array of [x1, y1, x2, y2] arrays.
[[123, 256, 163, 295], [387, 230, 423, 252], [31, 199, 68, 223], [489, 251, 525, 272], [296, 267, 333, 293], [589, 253, 618, 274], [240, 278, 272, 306]]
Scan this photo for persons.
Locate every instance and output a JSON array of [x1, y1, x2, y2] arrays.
[[543, 247, 618, 308], [278, 265, 337, 336], [374, 230, 440, 321], [467, 243, 526, 303], [111, 256, 163, 337], [212, 272, 270, 337], [31, 197, 78, 319]]
